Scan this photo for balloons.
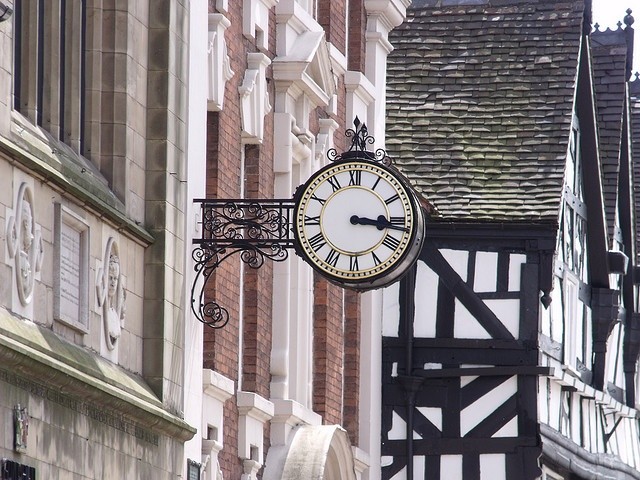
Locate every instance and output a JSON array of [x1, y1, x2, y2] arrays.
[[293, 152, 425, 293]]
